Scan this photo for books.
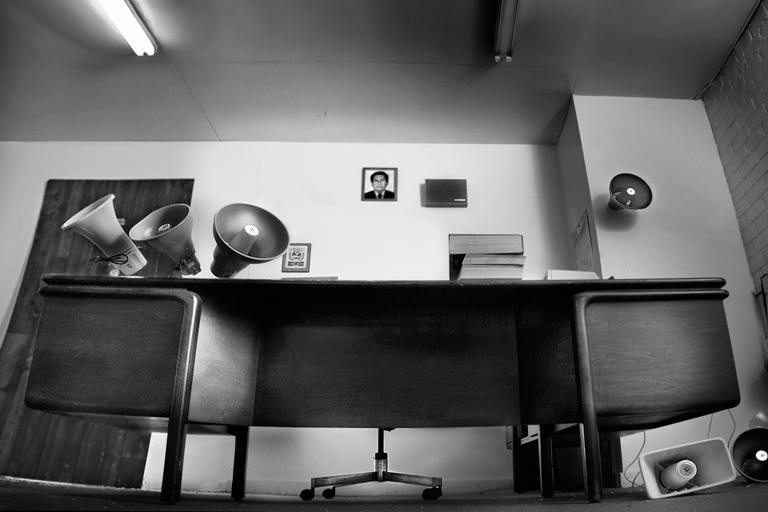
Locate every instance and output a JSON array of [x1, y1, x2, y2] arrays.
[[280, 275, 338, 280], [447, 232, 527, 281], [545, 268, 601, 280]]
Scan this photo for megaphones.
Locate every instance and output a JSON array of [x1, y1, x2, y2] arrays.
[[210, 203, 291, 281], [730, 427, 768, 484], [129, 203, 202, 276], [60, 193, 148, 276], [607, 172, 654, 212], [638, 437, 737, 500]]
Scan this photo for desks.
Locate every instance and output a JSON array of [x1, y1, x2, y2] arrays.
[[25, 273, 741, 504]]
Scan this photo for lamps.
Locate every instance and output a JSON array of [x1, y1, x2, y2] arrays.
[[492, 0, 522, 64], [95, 0, 158, 58]]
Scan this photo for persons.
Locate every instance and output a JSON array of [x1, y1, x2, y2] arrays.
[[364, 171, 395, 200]]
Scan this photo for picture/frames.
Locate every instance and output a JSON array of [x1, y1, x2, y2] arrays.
[[359, 167, 398, 201], [282, 242, 311, 273]]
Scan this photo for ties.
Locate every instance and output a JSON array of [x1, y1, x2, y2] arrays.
[[378, 194, 382, 199]]
